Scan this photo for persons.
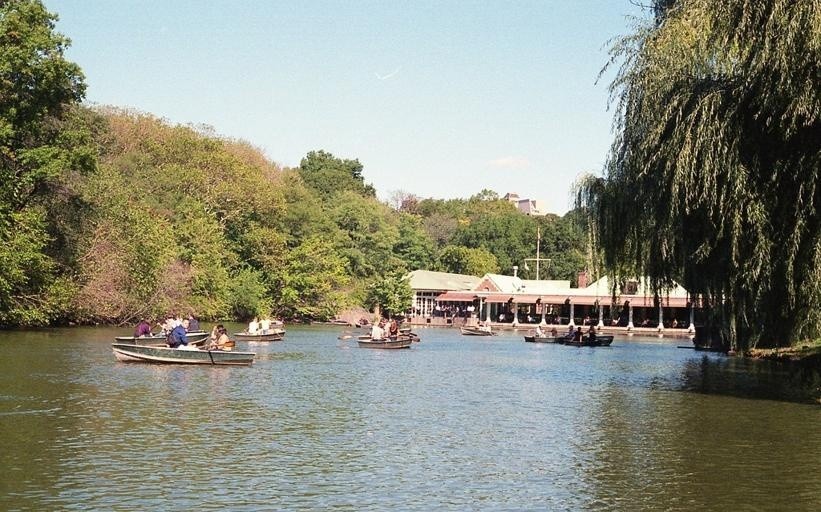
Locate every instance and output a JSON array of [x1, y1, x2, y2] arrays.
[[476, 322, 491, 332], [134, 315, 153, 338], [210, 325, 232, 351], [564, 325, 596, 342], [248, 316, 271, 334], [157, 313, 200, 348], [372, 318, 400, 340], [440, 304, 475, 322], [536, 326, 546, 338]]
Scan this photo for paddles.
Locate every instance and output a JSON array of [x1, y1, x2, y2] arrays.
[[390, 335, 420, 342], [198, 341, 235, 348], [338, 335, 372, 339]]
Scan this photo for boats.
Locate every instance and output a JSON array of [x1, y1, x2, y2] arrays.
[[357, 327, 412, 340], [109, 340, 257, 368], [356, 336, 414, 349], [559, 334, 614, 347], [233, 327, 286, 342], [115, 329, 211, 350], [523, 333, 565, 344], [459, 326, 505, 338]]
